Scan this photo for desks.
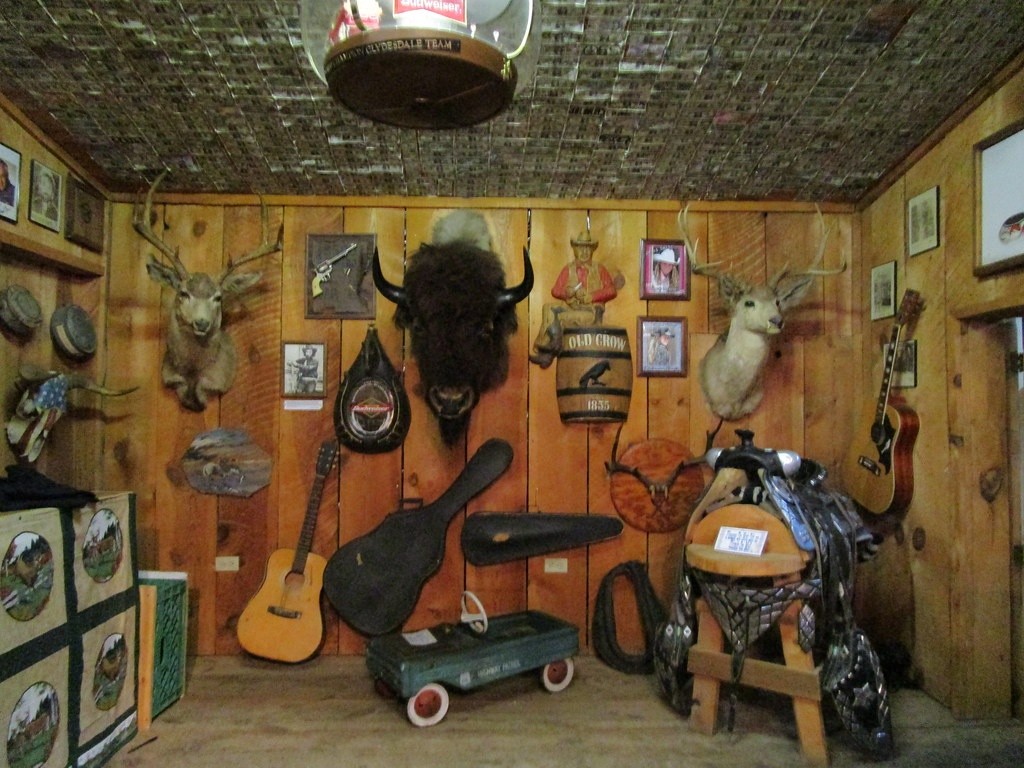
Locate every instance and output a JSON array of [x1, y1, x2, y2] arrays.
[[0, 490, 138, 768]]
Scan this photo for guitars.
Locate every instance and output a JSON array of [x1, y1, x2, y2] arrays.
[[841, 286, 922, 517], [237, 436, 342, 667]]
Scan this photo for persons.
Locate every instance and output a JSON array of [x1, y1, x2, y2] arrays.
[[895, 344, 914, 371], [33, 170, 57, 221], [648, 329, 676, 365], [529, 231, 617, 368], [0, 160, 15, 206], [288, 345, 319, 393], [652, 249, 681, 292]]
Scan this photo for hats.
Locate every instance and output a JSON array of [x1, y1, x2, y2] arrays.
[[653, 249, 681, 266], [651, 329, 677, 339]]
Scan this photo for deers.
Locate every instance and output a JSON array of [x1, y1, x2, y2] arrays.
[[676, 201, 847, 422], [132, 166, 284, 414]]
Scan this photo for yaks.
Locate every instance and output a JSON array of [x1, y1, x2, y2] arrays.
[[370, 239, 534, 452]]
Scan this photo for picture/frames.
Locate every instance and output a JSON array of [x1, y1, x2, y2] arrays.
[[908, 184, 940, 258], [870, 259, 897, 321], [28, 159, 63, 234], [972, 117, 1024, 278], [636, 315, 688, 379], [304, 232, 377, 321], [0, 142, 22, 225], [883, 339, 917, 389], [63, 175, 105, 255], [640, 238, 691, 302], [280, 339, 328, 399]]
